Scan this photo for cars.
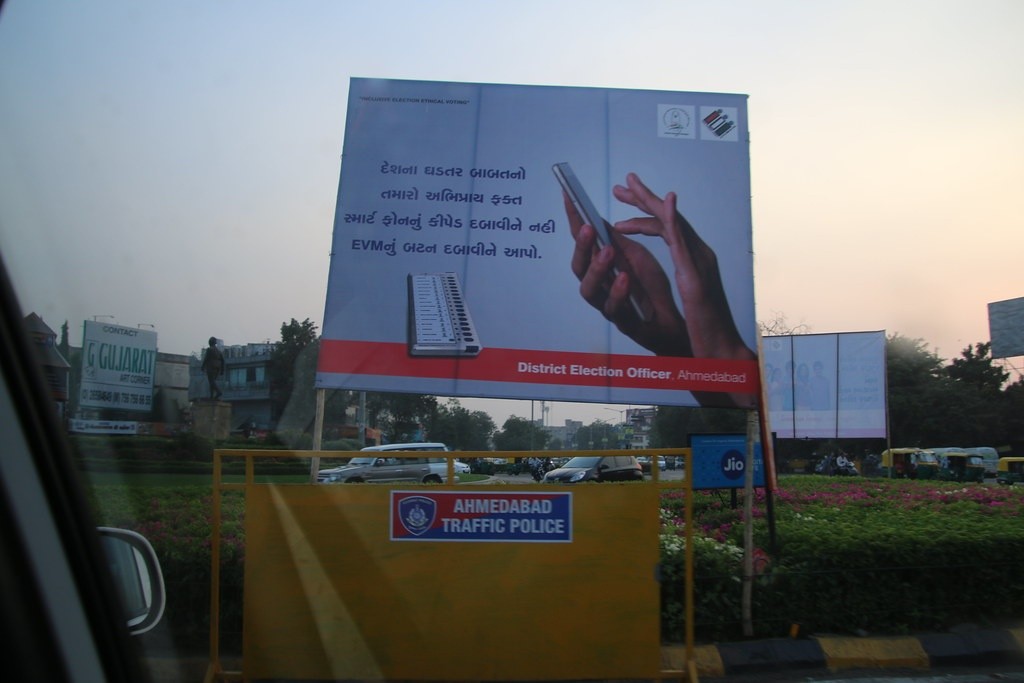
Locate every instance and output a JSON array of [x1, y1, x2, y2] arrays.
[[0, 258, 166, 683], [454, 456, 685, 485]]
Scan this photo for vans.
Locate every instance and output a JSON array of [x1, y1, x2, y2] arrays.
[[965, 447, 999, 476]]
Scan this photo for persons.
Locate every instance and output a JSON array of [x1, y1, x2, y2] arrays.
[[861, 448, 879, 477], [911, 455, 920, 480], [829, 450, 849, 475], [542, 457, 554, 472], [560, 171, 760, 409]]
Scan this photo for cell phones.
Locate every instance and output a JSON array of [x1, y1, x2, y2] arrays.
[[551, 161, 653, 323]]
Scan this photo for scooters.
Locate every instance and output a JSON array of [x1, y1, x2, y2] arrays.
[[834, 462, 860, 476], [529, 462, 544, 483]]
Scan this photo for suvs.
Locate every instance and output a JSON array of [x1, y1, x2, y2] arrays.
[[318, 443, 460, 484]]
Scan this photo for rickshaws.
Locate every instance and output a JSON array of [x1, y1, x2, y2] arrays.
[[854, 447, 984, 484], [996, 457, 1024, 485]]
